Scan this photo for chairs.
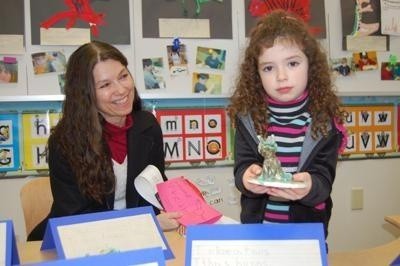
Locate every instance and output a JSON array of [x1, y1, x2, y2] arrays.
[[19, 178, 53, 239]]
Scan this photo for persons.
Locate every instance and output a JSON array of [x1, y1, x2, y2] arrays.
[[25, 39, 186, 242], [170, 46, 183, 65], [225, 9, 349, 256], [143, 58, 163, 89], [203, 48, 223, 70], [31, 52, 57, 74], [0, 62, 18, 83], [355, 52, 378, 71], [333, 58, 354, 78], [194, 73, 216, 95]]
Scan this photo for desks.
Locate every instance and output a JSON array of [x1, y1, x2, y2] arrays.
[[16, 213, 400, 266]]
